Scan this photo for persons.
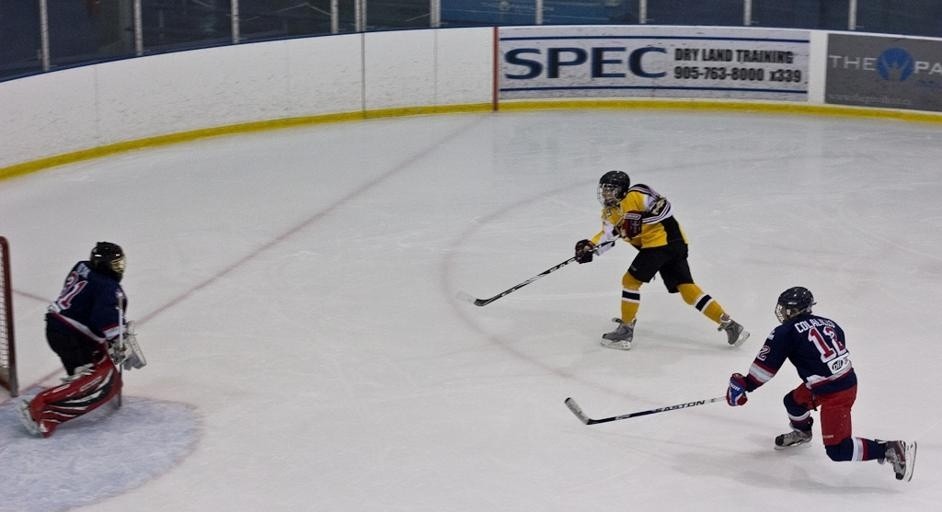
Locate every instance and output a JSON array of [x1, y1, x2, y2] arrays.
[[726, 286, 906, 482], [575, 169, 743, 346], [13, 239, 131, 437]]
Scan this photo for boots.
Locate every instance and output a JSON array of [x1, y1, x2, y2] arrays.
[[875, 438, 907, 479], [775, 418, 814, 446], [718, 314, 742, 344], [603, 317, 636, 342]]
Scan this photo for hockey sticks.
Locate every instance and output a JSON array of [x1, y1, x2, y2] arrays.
[[565, 397, 726, 424], [456, 235, 620, 307], [109, 291, 124, 408]]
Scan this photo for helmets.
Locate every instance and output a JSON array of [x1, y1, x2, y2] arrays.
[[775, 287, 813, 324], [90, 243, 125, 281], [597, 172, 629, 208]]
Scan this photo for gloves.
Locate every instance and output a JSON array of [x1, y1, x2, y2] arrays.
[[726, 373, 755, 406], [575, 240, 594, 263], [624, 212, 641, 236]]
